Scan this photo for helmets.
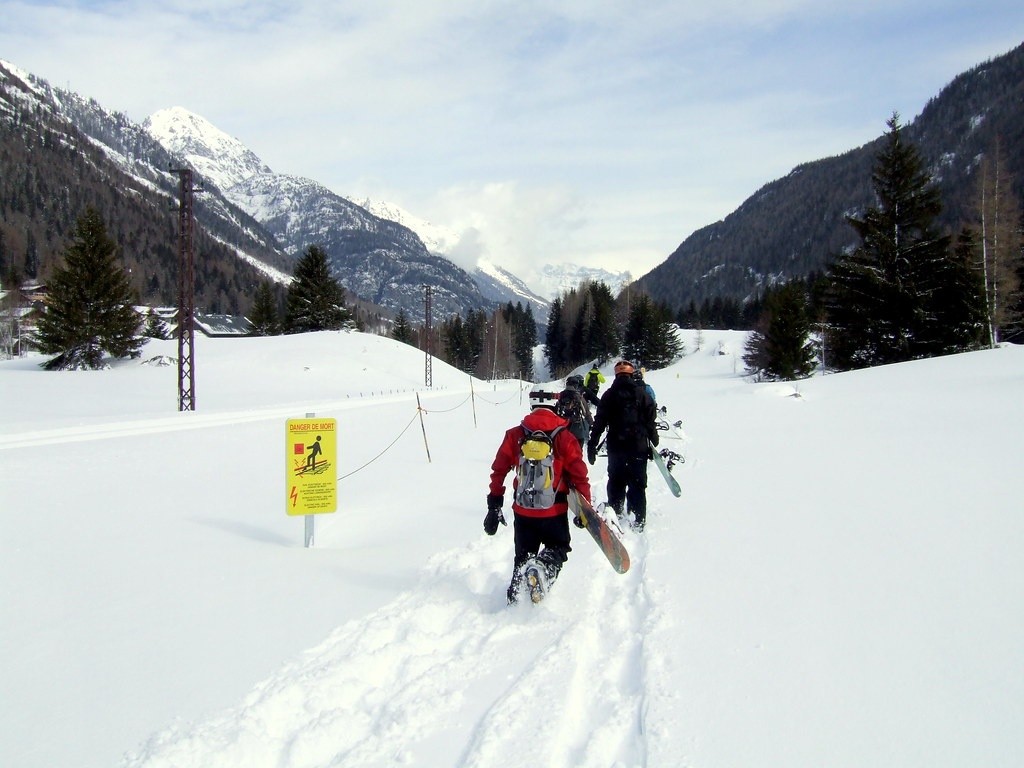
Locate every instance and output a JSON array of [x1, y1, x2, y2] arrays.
[[614, 361, 635, 375], [633, 370, 642, 379], [566, 377, 579, 389], [529, 383, 561, 406], [593, 364, 598, 369], [575, 375, 584, 382]]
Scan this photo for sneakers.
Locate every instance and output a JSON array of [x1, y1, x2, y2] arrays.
[[525, 565, 544, 603], [507, 601, 517, 607]]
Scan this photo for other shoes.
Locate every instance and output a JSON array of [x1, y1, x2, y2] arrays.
[[629, 522, 646, 533]]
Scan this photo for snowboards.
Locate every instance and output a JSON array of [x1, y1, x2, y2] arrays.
[[647, 438, 685, 498], [567, 485, 631, 574], [656, 406, 687, 439]]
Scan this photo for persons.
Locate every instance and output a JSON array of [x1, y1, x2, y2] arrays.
[[587, 360, 658, 533], [634, 370, 656, 401], [584, 363, 606, 405], [575, 374, 601, 410], [482, 383, 592, 609], [555, 375, 590, 454]]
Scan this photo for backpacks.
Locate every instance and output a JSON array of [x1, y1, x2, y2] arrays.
[[577, 386, 586, 397], [589, 372, 599, 390], [634, 380, 646, 391], [516, 422, 566, 510], [557, 390, 586, 423], [608, 388, 642, 449]]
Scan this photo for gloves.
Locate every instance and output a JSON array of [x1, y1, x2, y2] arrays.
[[649, 447, 653, 460], [573, 516, 584, 529], [484, 506, 507, 535], [588, 445, 597, 465]]
